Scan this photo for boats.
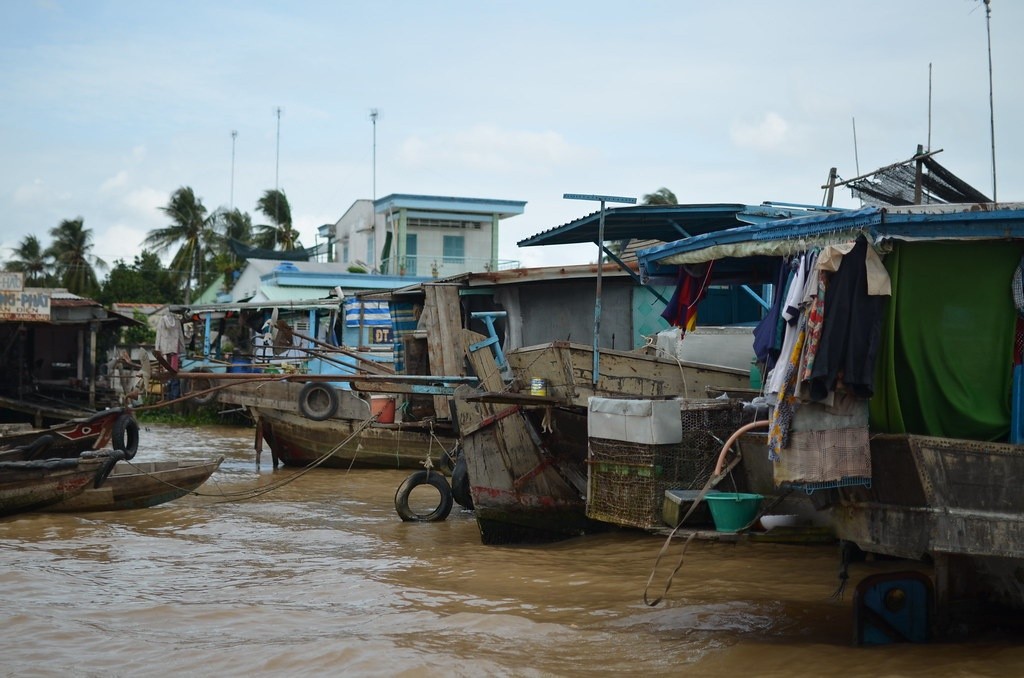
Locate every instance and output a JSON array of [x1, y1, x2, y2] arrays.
[[0, 200, 1023, 650]]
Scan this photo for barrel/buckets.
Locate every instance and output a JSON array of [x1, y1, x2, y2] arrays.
[[531, 377, 548, 397], [231, 357, 252, 373], [705, 491, 765, 533], [371, 394, 395, 424]]
